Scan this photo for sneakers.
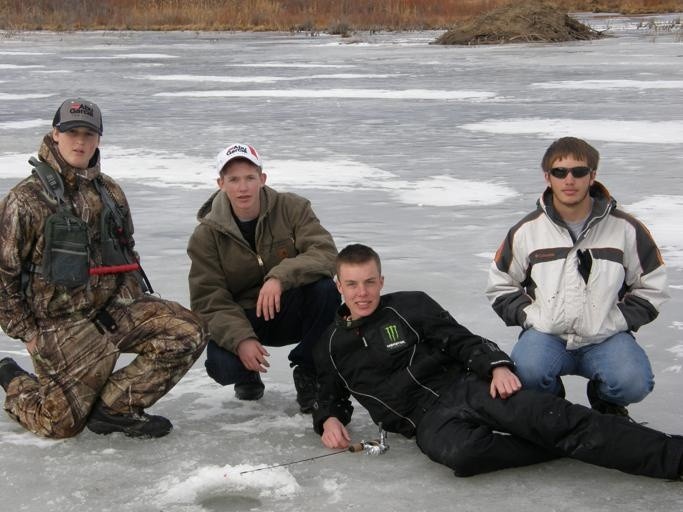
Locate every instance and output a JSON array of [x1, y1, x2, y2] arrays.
[[585, 379, 630, 423], [85, 395, 174, 440], [232, 371, 265, 402], [0, 356, 40, 396], [292, 365, 321, 415]]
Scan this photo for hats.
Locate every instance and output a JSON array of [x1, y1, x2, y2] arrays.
[[214, 141, 262, 172], [50, 97, 104, 138]]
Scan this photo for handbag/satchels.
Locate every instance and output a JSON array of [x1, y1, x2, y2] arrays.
[[40, 210, 93, 288]]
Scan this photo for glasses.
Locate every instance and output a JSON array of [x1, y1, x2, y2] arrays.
[[546, 166, 592, 180]]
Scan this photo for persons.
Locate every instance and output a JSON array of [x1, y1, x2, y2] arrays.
[[0, 99, 208, 438], [186, 140, 335, 411], [483, 136, 671, 418], [314, 241, 682, 482]]
